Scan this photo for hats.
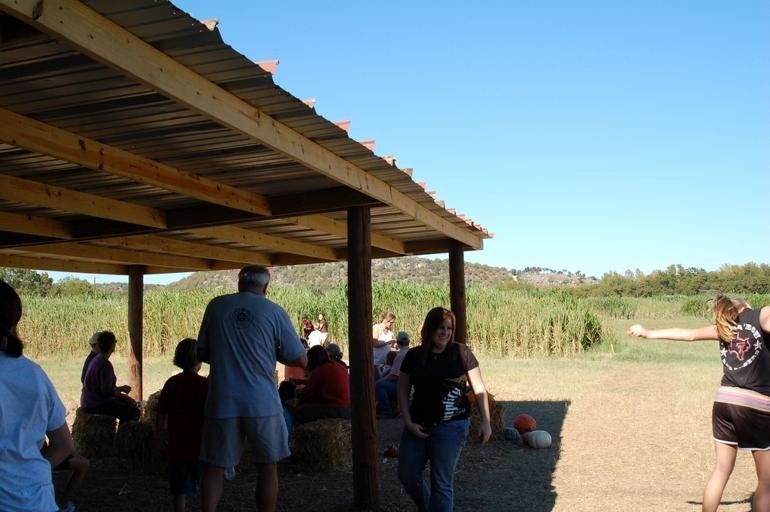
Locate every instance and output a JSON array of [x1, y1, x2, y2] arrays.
[[88, 332, 101, 344], [396, 332, 409, 343]]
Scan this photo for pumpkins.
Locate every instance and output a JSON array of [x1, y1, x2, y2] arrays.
[[527, 430, 552, 449], [514, 413, 537, 434]]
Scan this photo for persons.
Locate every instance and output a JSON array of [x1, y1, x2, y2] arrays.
[[1, 279, 76, 511], [373, 311, 399, 366], [375, 330, 411, 415], [153, 338, 210, 512], [326, 343, 348, 372], [80, 331, 143, 433], [375, 350, 396, 379], [315, 312, 329, 349], [286, 345, 351, 420], [283, 338, 310, 389], [625, 293, 770, 512], [80, 332, 103, 386], [396, 306, 492, 512], [195, 263, 312, 510], [301, 315, 314, 340]]
